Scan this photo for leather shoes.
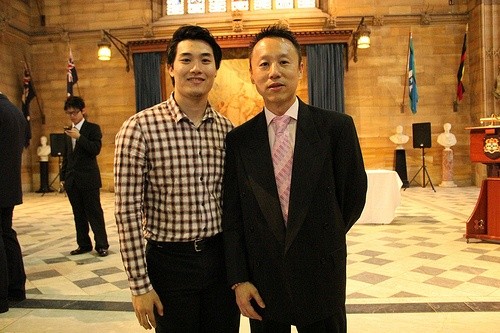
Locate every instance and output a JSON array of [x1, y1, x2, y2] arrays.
[[97, 247, 108, 256], [71, 247, 92, 255]]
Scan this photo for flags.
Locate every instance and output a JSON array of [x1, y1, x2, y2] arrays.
[[66, 48, 78, 99], [22, 67, 35, 124], [457, 33, 467, 101], [408, 36, 418, 113]]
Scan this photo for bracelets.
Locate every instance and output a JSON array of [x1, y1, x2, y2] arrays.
[[232, 283, 240, 290]]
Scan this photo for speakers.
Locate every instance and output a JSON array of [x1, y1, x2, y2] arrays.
[[412, 122, 431, 148], [49, 134, 63, 156]]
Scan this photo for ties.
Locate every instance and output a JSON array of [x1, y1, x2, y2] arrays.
[[271, 115, 293, 228]]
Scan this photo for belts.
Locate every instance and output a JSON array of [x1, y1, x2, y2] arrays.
[[148, 240, 206, 254]]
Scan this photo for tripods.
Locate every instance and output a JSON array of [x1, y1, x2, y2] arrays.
[[41, 157, 67, 197], [404, 148, 436, 192]]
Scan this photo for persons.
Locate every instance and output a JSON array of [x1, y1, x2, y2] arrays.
[[389, 125, 409, 149], [37, 136, 51, 161], [0, 92, 32, 315], [113, 25, 242, 333], [60, 96, 109, 256], [222, 22, 367, 333], [437, 123, 456, 150]]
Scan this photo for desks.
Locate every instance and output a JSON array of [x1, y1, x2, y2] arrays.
[[353, 169, 403, 225]]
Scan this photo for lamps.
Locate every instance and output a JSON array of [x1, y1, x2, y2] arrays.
[[97, 30, 133, 74], [351, 17, 371, 62]]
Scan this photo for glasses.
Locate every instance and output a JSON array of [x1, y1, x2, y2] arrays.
[[66, 110, 80, 116]]
